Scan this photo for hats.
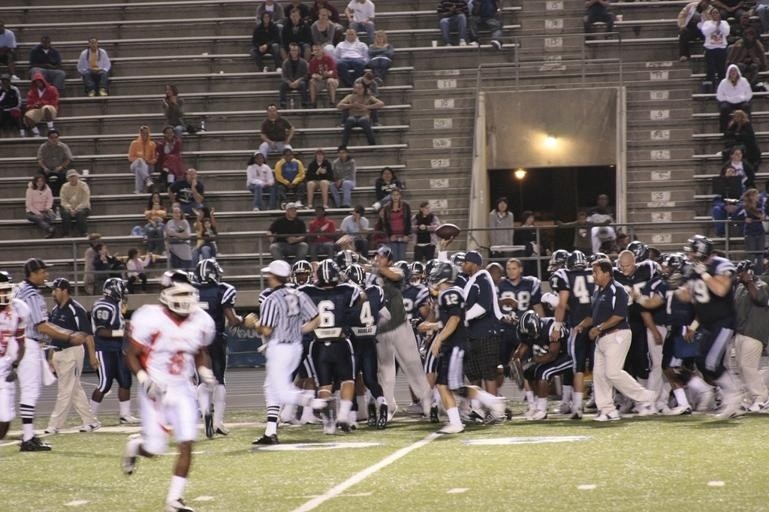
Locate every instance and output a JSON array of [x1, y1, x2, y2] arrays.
[[65, 169, 79, 178], [456, 250, 483, 265], [23, 257, 54, 273], [259, 259, 291, 278], [253, 151, 262, 159], [372, 246, 394, 263], [89, 232, 101, 243], [0, 73, 11, 80], [285, 202, 297, 210], [282, 144, 293, 152], [45, 277, 70, 290]]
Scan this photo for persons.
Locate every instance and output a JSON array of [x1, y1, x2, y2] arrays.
[[24, 174, 55, 237], [128, 125, 158, 193], [193, 205, 216, 230], [11, 258, 86, 451], [471, 0, 502, 49], [717, 65, 754, 130], [246, 154, 276, 213], [306, 148, 333, 212], [126, 249, 151, 293], [736, 28, 767, 76], [591, 193, 614, 218], [77, 37, 111, 96], [83, 233, 111, 300], [36, 128, 73, 185], [247, 249, 501, 445], [521, 211, 536, 228], [723, 109, 761, 168], [192, 218, 217, 263], [380, 188, 411, 252], [558, 212, 612, 248], [121, 270, 207, 512], [168, 168, 205, 214], [584, 0, 616, 42], [276, 149, 304, 208], [89, 278, 142, 425], [44, 277, 101, 436], [161, 84, 188, 135], [0, 271, 30, 440], [30, 35, 66, 90], [732, 10, 762, 35], [266, 202, 307, 261], [305, 208, 336, 262], [701, 8, 730, 85], [488, 198, 515, 246], [24, 72, 59, 138], [165, 207, 191, 269], [330, 146, 358, 209], [0, 21, 20, 81], [732, 188, 765, 274], [94, 244, 111, 276], [720, 146, 756, 190], [713, 165, 743, 232], [677, 0, 715, 63], [0, 77, 26, 138], [437, 0, 469, 48], [155, 126, 184, 182], [146, 203, 165, 227], [374, 167, 402, 208], [189, 260, 244, 437], [56, 169, 91, 236], [252, 0, 392, 156], [339, 207, 370, 242], [411, 201, 438, 255], [144, 192, 167, 241], [502, 238, 769, 424]]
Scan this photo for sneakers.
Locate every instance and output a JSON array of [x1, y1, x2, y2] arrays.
[[118, 415, 143, 425], [524, 407, 536, 418], [470, 409, 486, 425], [214, 421, 230, 436], [204, 411, 214, 439], [667, 403, 692, 416], [586, 397, 596, 407], [166, 496, 193, 512], [527, 408, 549, 420], [350, 419, 359, 430], [322, 418, 337, 435], [79, 420, 102, 433], [436, 421, 466, 434], [335, 416, 351, 433], [19, 436, 52, 452], [594, 408, 622, 422], [366, 399, 378, 427], [490, 394, 507, 421], [299, 415, 323, 426], [280, 411, 299, 425], [122, 432, 140, 474], [44, 426, 57, 433], [375, 401, 389, 430], [570, 406, 584, 419], [429, 402, 440, 423], [252, 432, 279, 445], [748, 398, 769, 413], [403, 402, 424, 415], [553, 401, 571, 414]]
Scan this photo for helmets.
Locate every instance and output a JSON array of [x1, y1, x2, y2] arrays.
[[425, 259, 445, 276], [292, 259, 314, 285], [567, 250, 588, 270], [317, 258, 341, 285], [194, 258, 225, 284], [666, 252, 688, 272], [336, 249, 359, 271], [590, 252, 610, 265], [547, 249, 569, 271], [517, 309, 541, 338], [0, 270, 14, 309], [682, 235, 714, 256], [626, 240, 649, 263], [408, 260, 424, 285], [102, 277, 125, 302], [435, 262, 458, 283], [158, 270, 201, 315], [345, 264, 367, 288], [186, 271, 200, 283]]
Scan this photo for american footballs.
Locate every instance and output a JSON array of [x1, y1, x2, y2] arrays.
[[434, 224, 460, 239]]
